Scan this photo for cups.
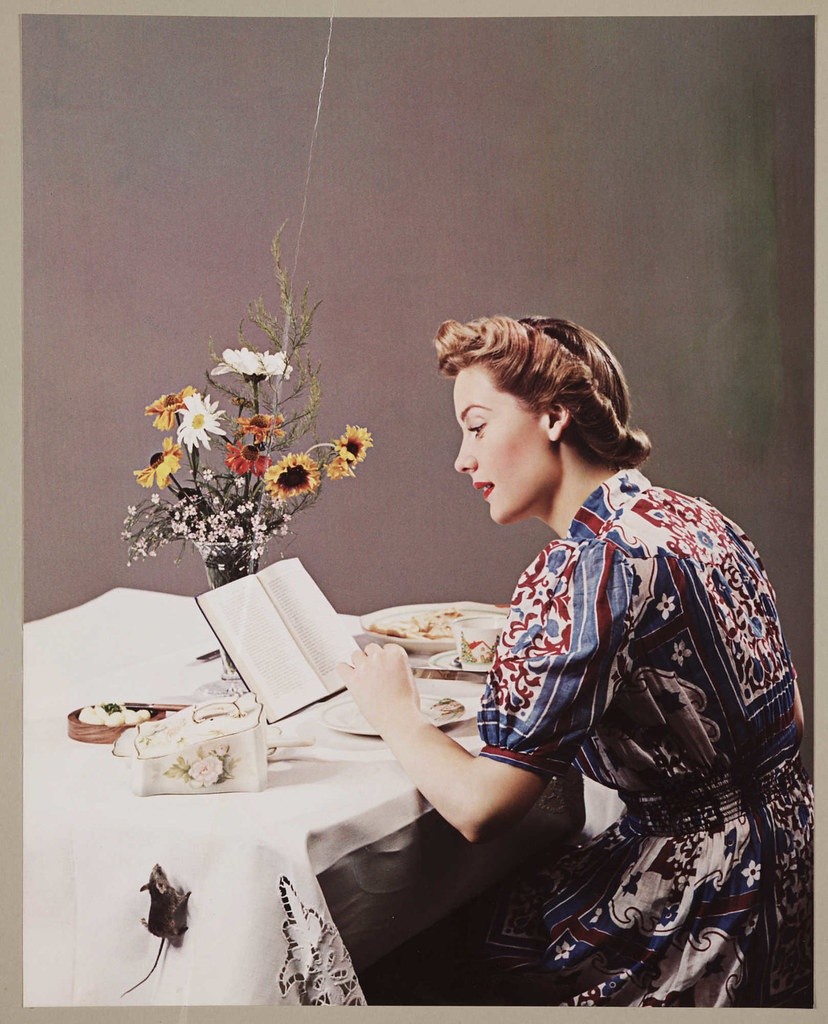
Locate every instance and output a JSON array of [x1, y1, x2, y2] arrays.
[[452, 615, 508, 670], [426, 649, 509, 670]]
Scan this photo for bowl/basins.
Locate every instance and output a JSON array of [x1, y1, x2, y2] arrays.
[[358, 602, 510, 656]]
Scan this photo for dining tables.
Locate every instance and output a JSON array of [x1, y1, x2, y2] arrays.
[[23, 587, 625, 1007]]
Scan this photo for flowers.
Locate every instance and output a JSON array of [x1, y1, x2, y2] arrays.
[[119, 219, 374, 575]]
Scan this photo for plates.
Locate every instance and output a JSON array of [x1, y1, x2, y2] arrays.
[[319, 693, 465, 738]]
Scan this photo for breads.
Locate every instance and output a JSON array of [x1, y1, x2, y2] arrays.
[[371, 609, 465, 640]]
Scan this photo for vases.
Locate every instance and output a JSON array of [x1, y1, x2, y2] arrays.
[[194, 543, 265, 680]]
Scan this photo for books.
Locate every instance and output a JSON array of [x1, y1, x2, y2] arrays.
[[196, 555, 365, 725]]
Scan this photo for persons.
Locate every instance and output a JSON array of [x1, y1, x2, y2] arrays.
[[340, 310, 813, 1006]]
